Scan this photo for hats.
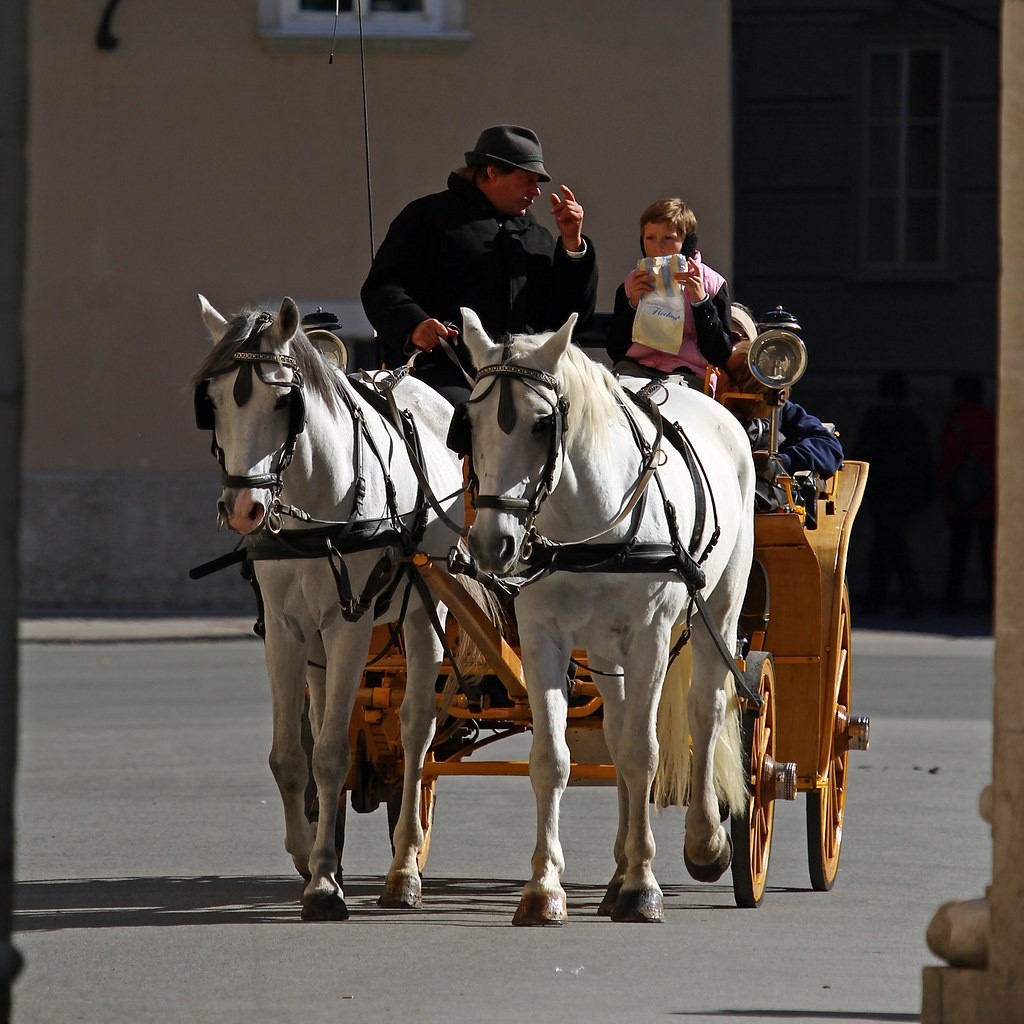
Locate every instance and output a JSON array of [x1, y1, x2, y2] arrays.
[[464, 124, 552, 182]]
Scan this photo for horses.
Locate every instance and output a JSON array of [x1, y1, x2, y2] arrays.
[[194, 292, 528, 921], [461, 305, 756, 926]]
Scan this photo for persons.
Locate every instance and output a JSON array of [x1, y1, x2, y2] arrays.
[[728, 340, 843, 510], [605, 197, 734, 392], [359, 126, 599, 458]]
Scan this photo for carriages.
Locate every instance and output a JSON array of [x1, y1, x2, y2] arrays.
[[194, 291, 874, 927]]
